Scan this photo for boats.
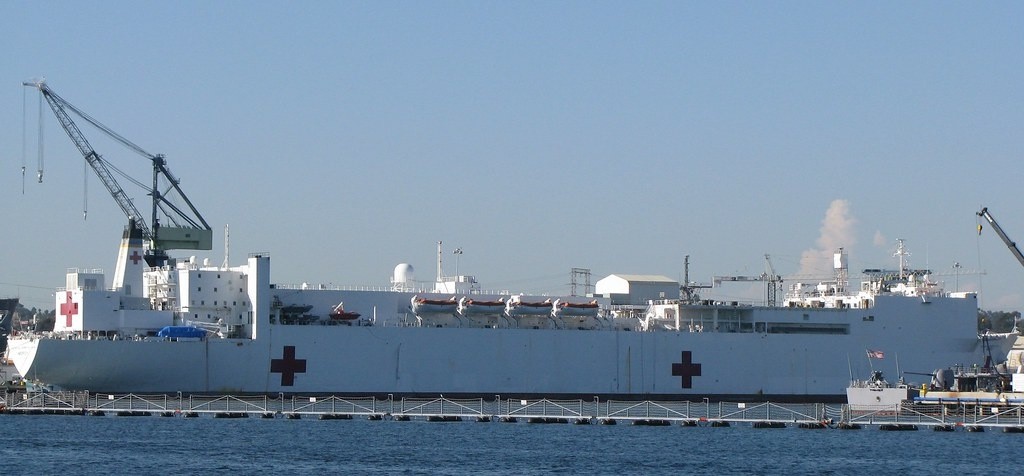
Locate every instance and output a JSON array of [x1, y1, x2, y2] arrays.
[[845, 346, 918, 416], [914, 352, 1023, 414]]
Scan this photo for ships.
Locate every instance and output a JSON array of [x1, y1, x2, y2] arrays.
[[5, 215, 1019, 404]]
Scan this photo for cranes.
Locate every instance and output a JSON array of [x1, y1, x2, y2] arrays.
[[18, 75, 214, 269], [713, 253, 783, 307]]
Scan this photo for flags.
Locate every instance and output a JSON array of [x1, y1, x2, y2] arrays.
[[865, 347, 884, 358]]
